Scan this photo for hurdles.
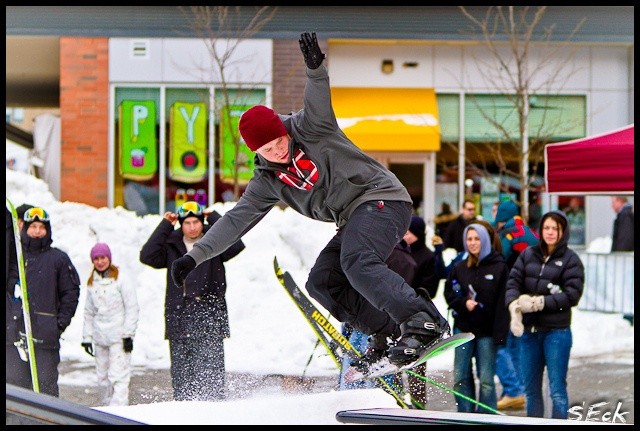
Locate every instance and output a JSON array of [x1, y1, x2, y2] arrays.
[[342, 332, 475, 383]]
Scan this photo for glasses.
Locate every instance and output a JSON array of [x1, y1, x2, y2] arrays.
[[464, 207, 475, 211], [178, 202, 202, 217], [24, 208, 49, 222]]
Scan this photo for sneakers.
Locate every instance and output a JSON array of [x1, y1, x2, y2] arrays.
[[350, 328, 401, 372], [497, 395, 524, 410], [388, 316, 446, 364]]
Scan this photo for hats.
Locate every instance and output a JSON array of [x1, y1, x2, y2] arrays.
[[239, 106, 288, 152], [91, 243, 111, 263], [15, 204, 33, 217], [496, 199, 518, 222], [177, 211, 203, 224]]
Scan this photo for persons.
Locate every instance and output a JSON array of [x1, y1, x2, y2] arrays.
[[139, 199, 249, 403], [608, 194, 634, 252], [504, 210, 585, 420], [329, 321, 395, 392], [562, 197, 586, 246], [441, 198, 495, 253], [79, 240, 139, 406], [490, 201, 500, 224], [396, 213, 444, 410], [5, 205, 82, 399], [171, 31, 446, 373], [15, 203, 35, 233], [430, 222, 506, 412], [492, 200, 539, 410], [434, 200, 458, 237]]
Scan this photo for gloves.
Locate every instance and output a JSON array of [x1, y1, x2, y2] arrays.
[[297, 32, 325, 69], [124, 338, 133, 352], [83, 343, 94, 355], [508, 300, 524, 337], [517, 294, 544, 314], [172, 255, 195, 288]]
